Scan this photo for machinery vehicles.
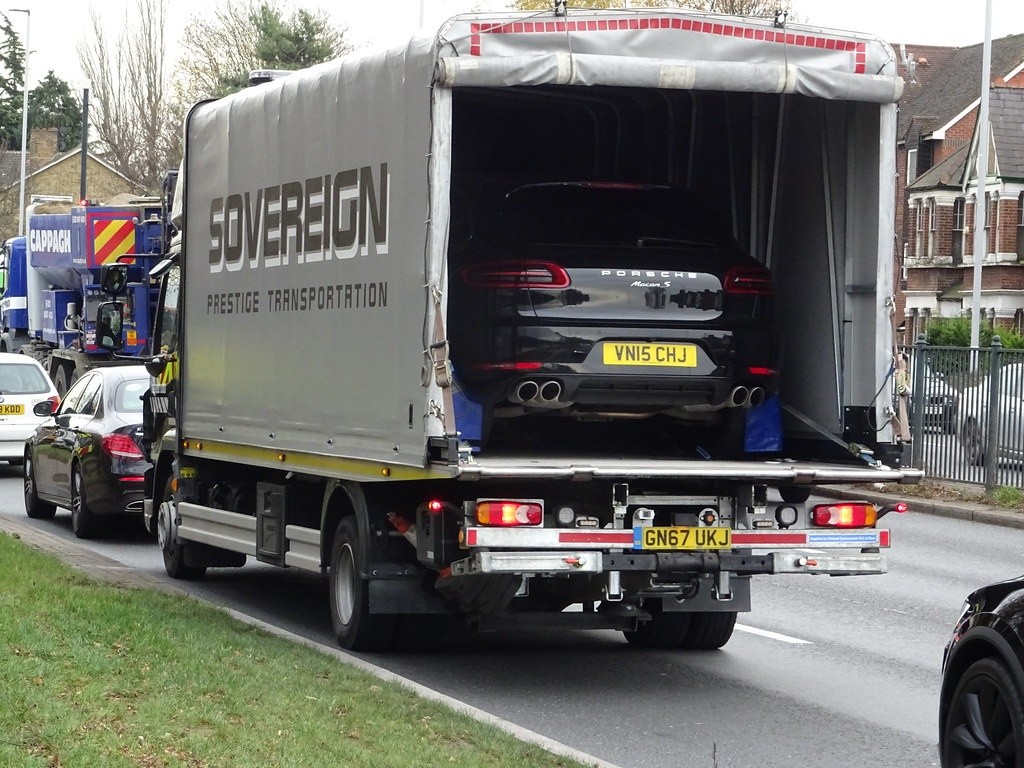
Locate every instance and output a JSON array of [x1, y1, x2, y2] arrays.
[[0, 194, 171, 397]]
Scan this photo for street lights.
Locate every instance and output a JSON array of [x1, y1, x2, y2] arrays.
[[7, 6, 39, 230]]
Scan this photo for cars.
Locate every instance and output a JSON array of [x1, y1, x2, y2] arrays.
[[901, 352, 960, 435], [0, 350, 62, 474], [949, 362, 1024, 478], [460, 180, 780, 451], [935, 576, 1024, 767], [23, 365, 164, 538]]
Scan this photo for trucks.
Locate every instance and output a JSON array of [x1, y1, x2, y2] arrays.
[[91, 0, 908, 654]]
